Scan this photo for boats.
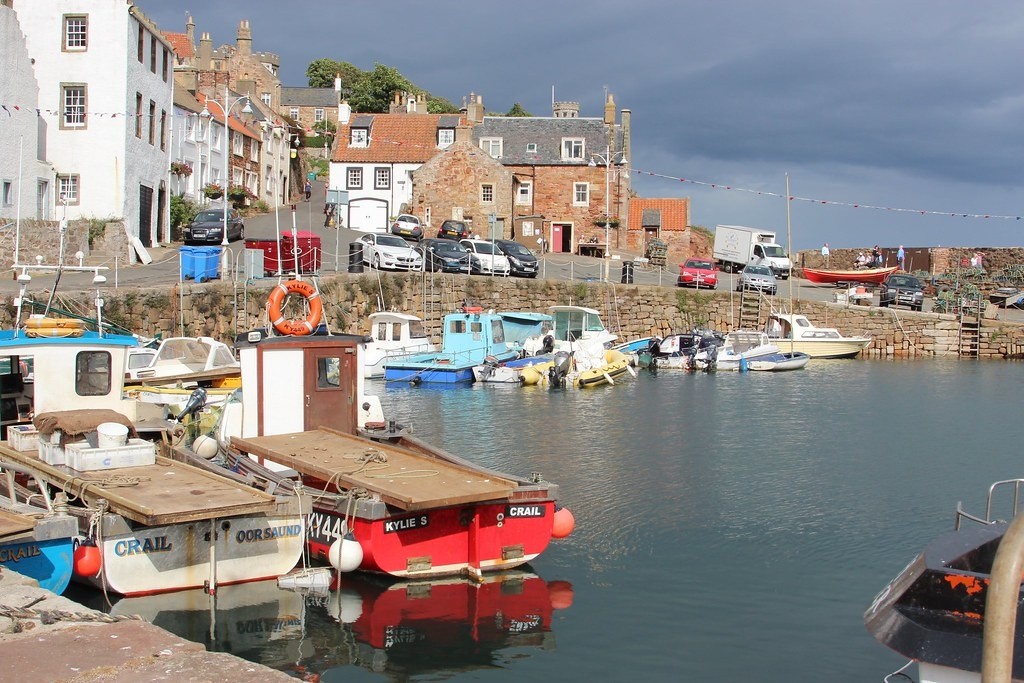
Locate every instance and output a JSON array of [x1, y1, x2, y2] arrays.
[[71, 580, 311, 658], [0, 460, 84, 596], [862, 479, 1024, 683], [803, 262, 902, 284], [0, 406, 331, 599], [730, 313, 873, 361], [0, 174, 242, 436], [612, 334, 663, 366], [155, 204, 574, 584], [657, 176, 812, 373], [291, 558, 576, 679], [323, 301, 633, 391]]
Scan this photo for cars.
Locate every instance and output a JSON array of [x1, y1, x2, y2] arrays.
[[736, 264, 778, 296], [391, 214, 427, 243], [487, 238, 540, 279], [677, 256, 720, 290], [437, 220, 468, 240], [413, 237, 482, 277], [452, 238, 511, 277], [354, 231, 423, 273], [182, 206, 245, 243]]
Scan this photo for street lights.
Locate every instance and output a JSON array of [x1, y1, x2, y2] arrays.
[[588, 143, 630, 283], [199, 91, 252, 280]]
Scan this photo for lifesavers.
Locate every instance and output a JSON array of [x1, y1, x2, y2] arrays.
[[268, 280, 323, 335], [463, 307, 482, 313], [23, 317, 87, 337]]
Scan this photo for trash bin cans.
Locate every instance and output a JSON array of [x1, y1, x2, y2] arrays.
[[193, 249, 211, 283], [308, 173, 316, 181], [348, 242, 364, 273], [281, 231, 321, 274], [243, 237, 282, 277], [179, 246, 198, 280], [621, 261, 634, 283], [198, 246, 222, 279]]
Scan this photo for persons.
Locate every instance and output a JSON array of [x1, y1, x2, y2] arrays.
[[302, 178, 311, 201], [578, 234, 584, 255], [589, 235, 599, 257], [970, 251, 985, 268], [851, 244, 881, 270], [323, 203, 335, 228], [896, 244, 905, 269], [821, 242, 829, 268], [648, 335, 659, 356]]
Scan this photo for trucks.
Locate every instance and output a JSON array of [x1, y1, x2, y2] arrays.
[[713, 225, 794, 280]]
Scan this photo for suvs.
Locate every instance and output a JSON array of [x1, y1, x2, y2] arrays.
[[879, 273, 925, 312]]
